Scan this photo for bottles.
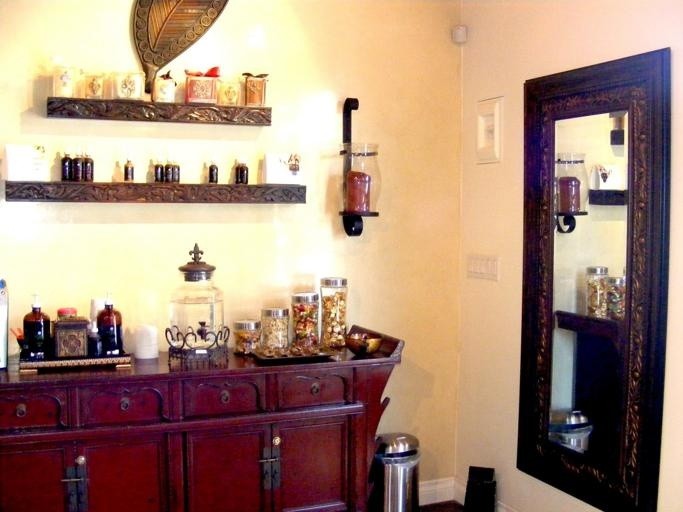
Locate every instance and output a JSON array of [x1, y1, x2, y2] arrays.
[[123, 157, 134, 183], [235, 159, 248, 184], [207, 159, 218, 184], [61, 150, 94, 182], [24, 298, 49, 358], [153, 156, 180, 184], [97, 296, 123, 353]]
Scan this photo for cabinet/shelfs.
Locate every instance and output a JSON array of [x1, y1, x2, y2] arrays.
[[183, 368, 365, 512], [0, 383, 188, 512], [555, 309, 623, 488]]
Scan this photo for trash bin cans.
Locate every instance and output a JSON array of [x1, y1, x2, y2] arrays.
[[376, 432, 419, 512], [549, 409, 593, 455]]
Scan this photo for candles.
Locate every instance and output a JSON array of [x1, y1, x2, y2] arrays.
[[559, 176, 580, 213]]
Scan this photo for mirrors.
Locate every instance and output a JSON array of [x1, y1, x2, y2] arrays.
[[515, 46, 672, 512]]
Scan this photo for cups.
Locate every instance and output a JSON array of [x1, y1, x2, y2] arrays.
[[558, 175, 583, 215]]
